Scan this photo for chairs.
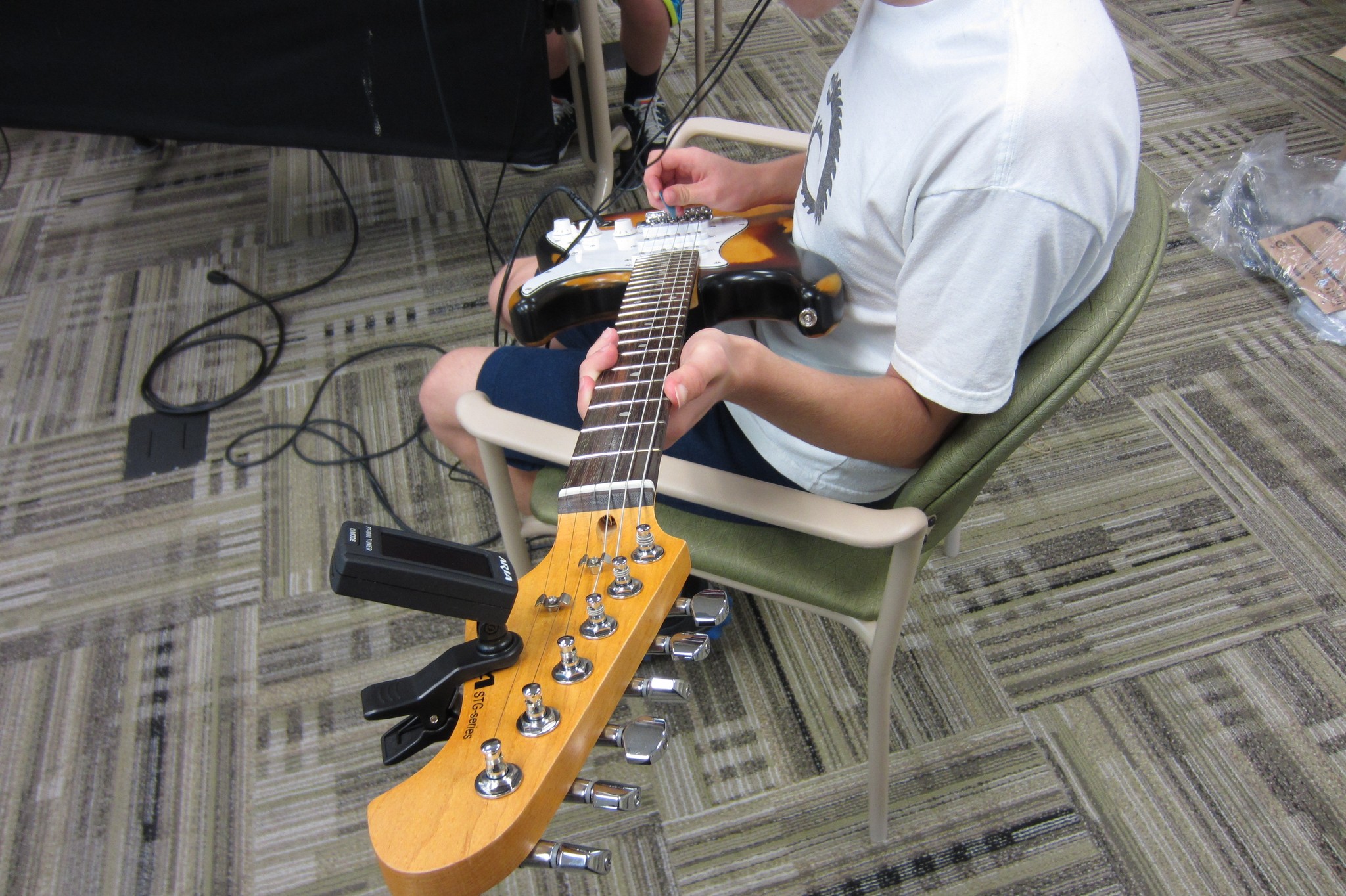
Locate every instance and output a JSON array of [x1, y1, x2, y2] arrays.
[[454, 0, 1168, 849]]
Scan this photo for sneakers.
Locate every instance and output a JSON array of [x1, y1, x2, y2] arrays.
[[622, 93, 673, 171], [510, 94, 577, 171]]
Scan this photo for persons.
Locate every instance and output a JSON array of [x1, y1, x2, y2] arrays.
[[510, 1, 685, 175], [418, 0, 1138, 655]]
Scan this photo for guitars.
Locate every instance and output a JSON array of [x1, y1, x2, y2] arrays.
[[363, 199, 851, 896]]
